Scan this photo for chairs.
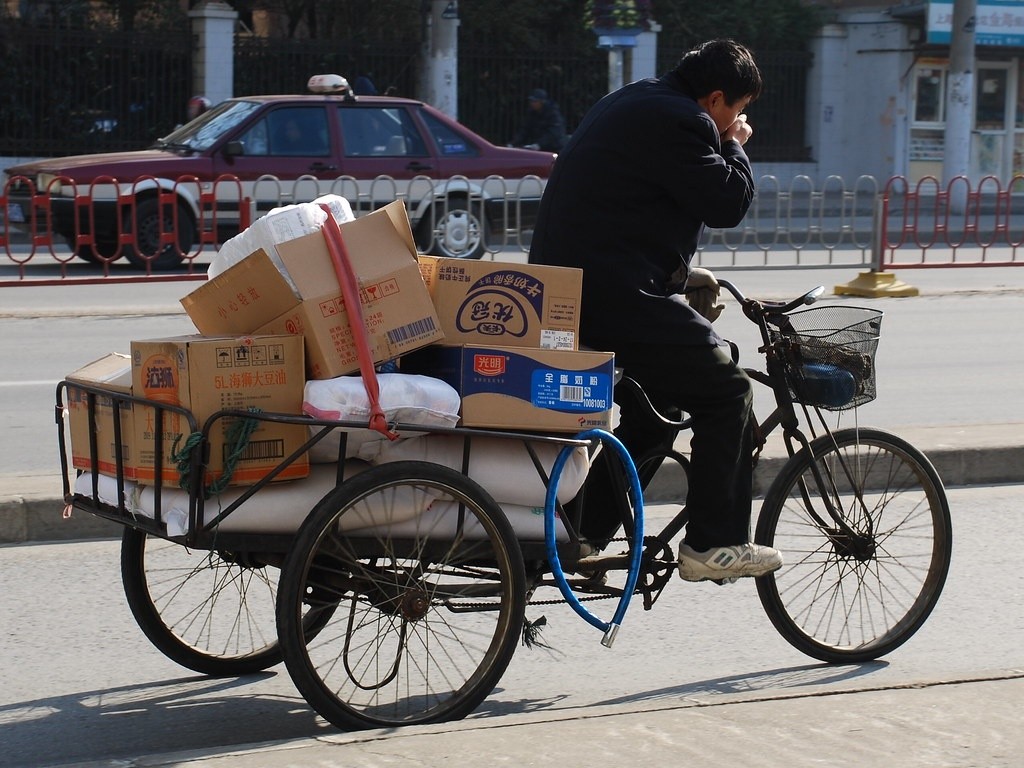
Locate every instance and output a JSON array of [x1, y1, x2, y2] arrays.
[[385, 135, 406, 155]]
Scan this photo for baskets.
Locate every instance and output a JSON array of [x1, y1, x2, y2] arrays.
[[765, 306, 884, 411]]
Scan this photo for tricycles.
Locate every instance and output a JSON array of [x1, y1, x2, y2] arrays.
[[54, 284, 955, 730]]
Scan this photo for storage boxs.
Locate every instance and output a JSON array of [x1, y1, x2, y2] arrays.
[[62, 199, 616, 489]]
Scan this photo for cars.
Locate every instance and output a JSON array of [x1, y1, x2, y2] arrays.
[[3, 73, 560, 271]]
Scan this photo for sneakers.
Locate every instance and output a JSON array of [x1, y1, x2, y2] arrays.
[[678, 538, 783, 582], [576, 536, 608, 583]]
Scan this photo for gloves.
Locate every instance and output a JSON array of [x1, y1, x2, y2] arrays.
[[686, 287, 726, 323], [687, 268, 720, 296]]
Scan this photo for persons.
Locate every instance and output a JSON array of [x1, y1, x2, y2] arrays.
[[175, 96, 210, 130], [527, 38, 782, 582], [505, 89, 569, 153]]
